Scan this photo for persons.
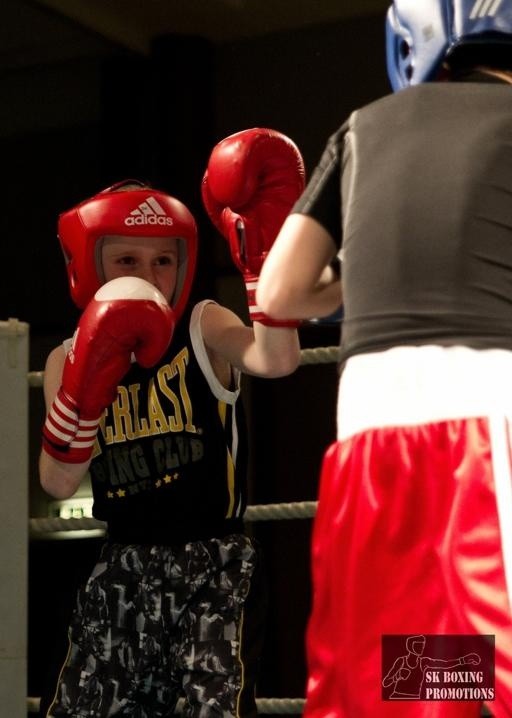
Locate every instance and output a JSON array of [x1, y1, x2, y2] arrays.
[[38, 126, 307, 718], [255, 0, 511, 718]]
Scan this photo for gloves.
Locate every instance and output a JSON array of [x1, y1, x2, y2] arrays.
[[40, 278, 175, 463], [202, 127, 307, 327]]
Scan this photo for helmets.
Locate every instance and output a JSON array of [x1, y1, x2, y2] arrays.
[[386, 0, 512, 92], [59, 179, 199, 320]]
